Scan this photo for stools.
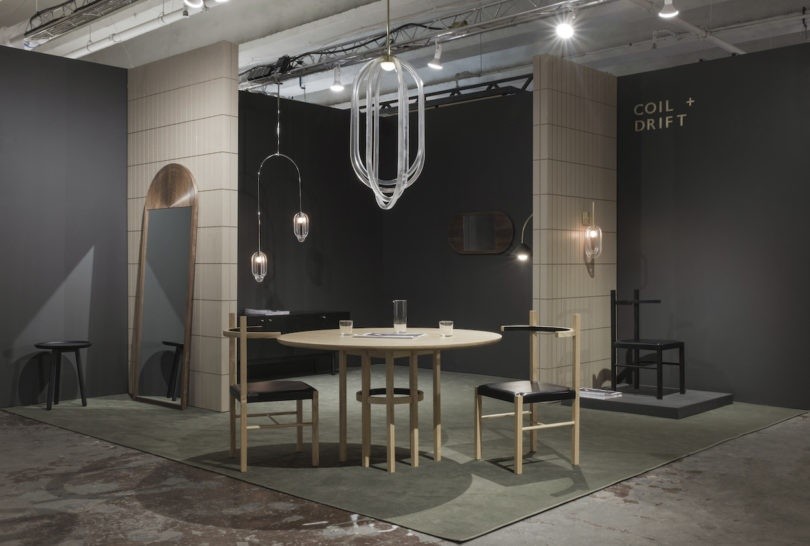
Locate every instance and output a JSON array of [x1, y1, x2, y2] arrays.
[[162, 340, 184, 401], [34, 341, 93, 411]]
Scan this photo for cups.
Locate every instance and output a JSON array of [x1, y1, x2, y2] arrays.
[[338, 320, 353, 335], [439, 321, 453, 337], [393, 300, 407, 333]]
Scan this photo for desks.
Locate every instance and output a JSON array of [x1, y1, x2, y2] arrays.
[[277, 328, 503, 473]]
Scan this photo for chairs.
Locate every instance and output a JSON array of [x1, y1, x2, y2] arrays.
[[475, 310, 580, 475], [610, 289, 686, 400], [222, 313, 318, 472]]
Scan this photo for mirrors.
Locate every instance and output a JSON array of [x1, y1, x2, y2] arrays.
[[138, 207, 193, 404]]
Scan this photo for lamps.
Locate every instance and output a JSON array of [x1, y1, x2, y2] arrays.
[[427, 38, 444, 70], [582, 200, 602, 258], [658, 0, 679, 19], [510, 214, 534, 261], [331, 65, 345, 91], [350, 0, 426, 209], [252, 81, 310, 284]]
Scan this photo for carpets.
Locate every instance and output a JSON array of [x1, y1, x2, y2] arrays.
[[0, 361, 809, 543]]
